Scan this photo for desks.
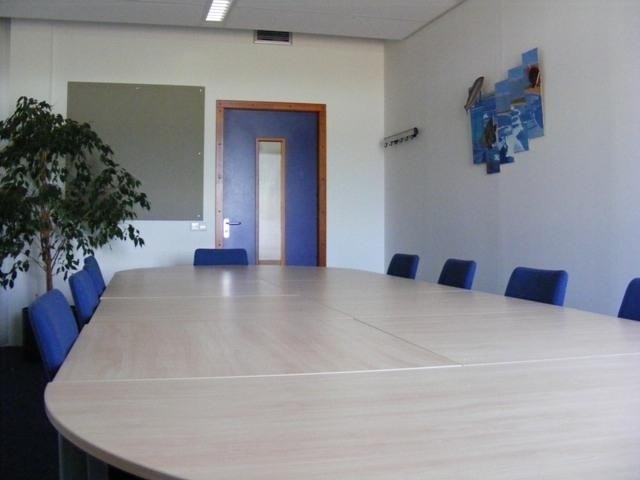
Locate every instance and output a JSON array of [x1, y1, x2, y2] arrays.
[[44, 265, 640, 480]]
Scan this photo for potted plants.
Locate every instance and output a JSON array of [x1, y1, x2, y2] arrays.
[[0, 96, 152, 364]]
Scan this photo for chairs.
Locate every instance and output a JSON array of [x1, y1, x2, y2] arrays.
[[68, 270, 99, 326], [387, 253, 420, 279], [194, 248, 249, 266], [504, 266, 568, 306], [82, 255, 106, 296], [617, 277, 640, 321], [29, 288, 79, 380], [437, 258, 477, 290]]
